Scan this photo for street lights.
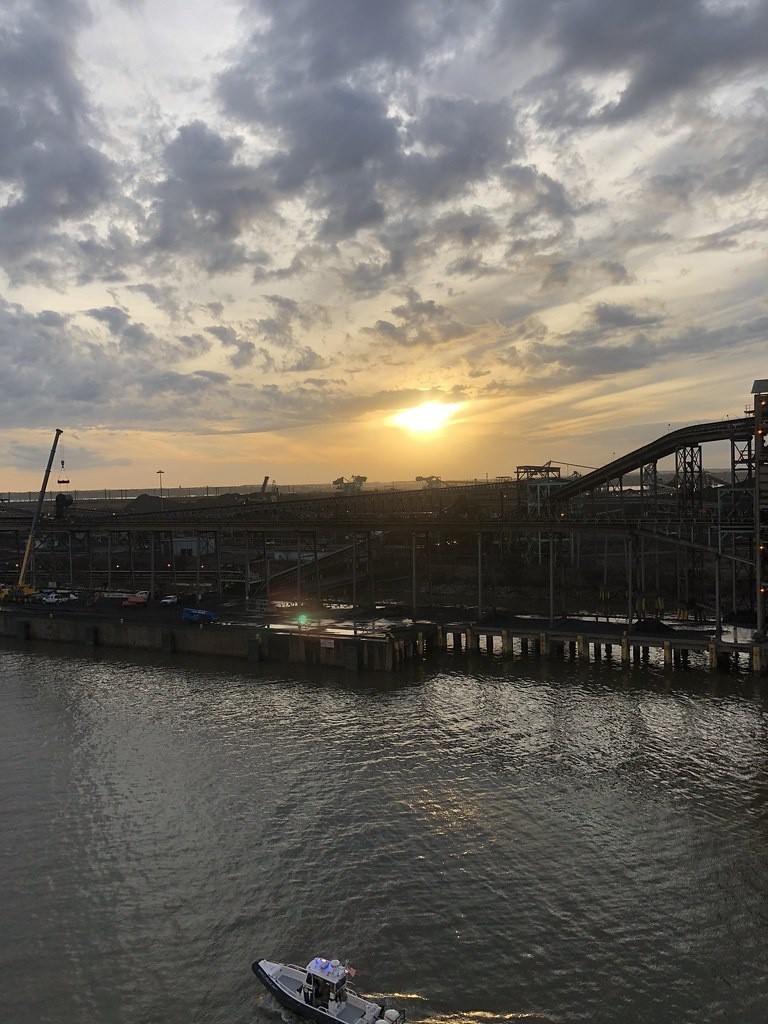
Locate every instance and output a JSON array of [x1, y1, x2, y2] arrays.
[[156, 470, 164, 511]]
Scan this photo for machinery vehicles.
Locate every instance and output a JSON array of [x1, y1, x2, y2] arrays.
[[0, 428, 71, 603]]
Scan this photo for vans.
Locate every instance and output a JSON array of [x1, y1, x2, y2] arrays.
[[264, 538, 281, 547]]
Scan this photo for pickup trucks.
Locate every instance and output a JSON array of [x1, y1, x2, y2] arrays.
[[42, 592, 69, 604]]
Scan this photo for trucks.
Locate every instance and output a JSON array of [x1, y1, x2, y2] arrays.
[[61, 584, 179, 611]]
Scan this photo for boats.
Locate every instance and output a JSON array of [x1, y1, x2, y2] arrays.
[[252, 956, 407, 1023]]
